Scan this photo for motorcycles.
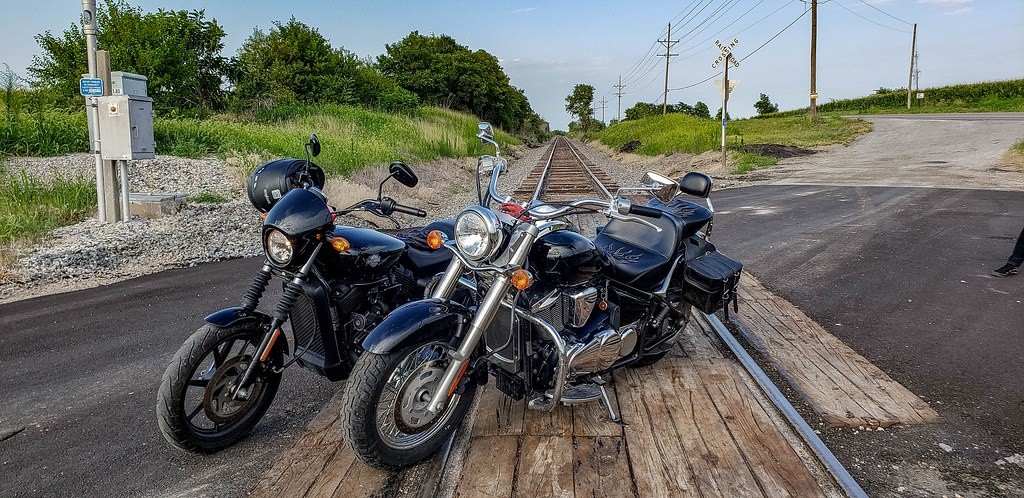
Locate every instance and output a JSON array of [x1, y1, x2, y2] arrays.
[[339, 121, 743, 472], [155, 133, 485, 455]]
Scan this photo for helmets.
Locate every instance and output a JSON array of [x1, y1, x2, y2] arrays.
[[246, 159, 325, 214]]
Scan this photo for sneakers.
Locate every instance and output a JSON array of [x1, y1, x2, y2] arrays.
[[991, 263, 1018, 277]]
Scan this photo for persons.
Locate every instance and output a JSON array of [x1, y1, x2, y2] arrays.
[[991, 227, 1024, 278]]
